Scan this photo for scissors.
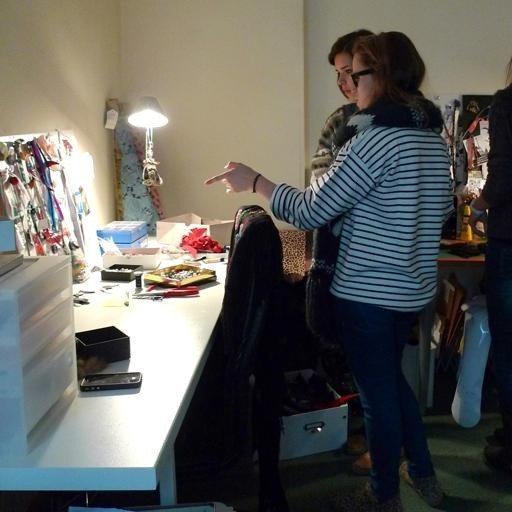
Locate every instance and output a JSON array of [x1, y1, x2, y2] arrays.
[[132, 284, 200, 298]]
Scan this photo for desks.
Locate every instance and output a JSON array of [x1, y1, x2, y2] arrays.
[[414, 244, 489, 419], [2, 249, 233, 511]]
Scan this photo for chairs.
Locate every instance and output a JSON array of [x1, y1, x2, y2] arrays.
[[178, 198, 293, 507]]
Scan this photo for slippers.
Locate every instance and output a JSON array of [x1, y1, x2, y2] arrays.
[[346, 435, 372, 475], [331, 484, 401, 511], [399, 460, 443, 508]]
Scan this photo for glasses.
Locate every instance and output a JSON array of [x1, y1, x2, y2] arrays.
[[350, 69, 374, 87]]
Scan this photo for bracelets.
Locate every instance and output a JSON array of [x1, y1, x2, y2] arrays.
[[470, 199, 486, 217]]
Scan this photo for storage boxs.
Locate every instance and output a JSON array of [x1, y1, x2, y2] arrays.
[[279, 366, 354, 465]]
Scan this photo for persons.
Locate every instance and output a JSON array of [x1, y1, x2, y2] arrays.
[[458, 101, 480, 136], [468, 58, 512, 468]]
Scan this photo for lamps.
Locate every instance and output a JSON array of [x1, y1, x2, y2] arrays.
[[126, 91, 170, 189]]
